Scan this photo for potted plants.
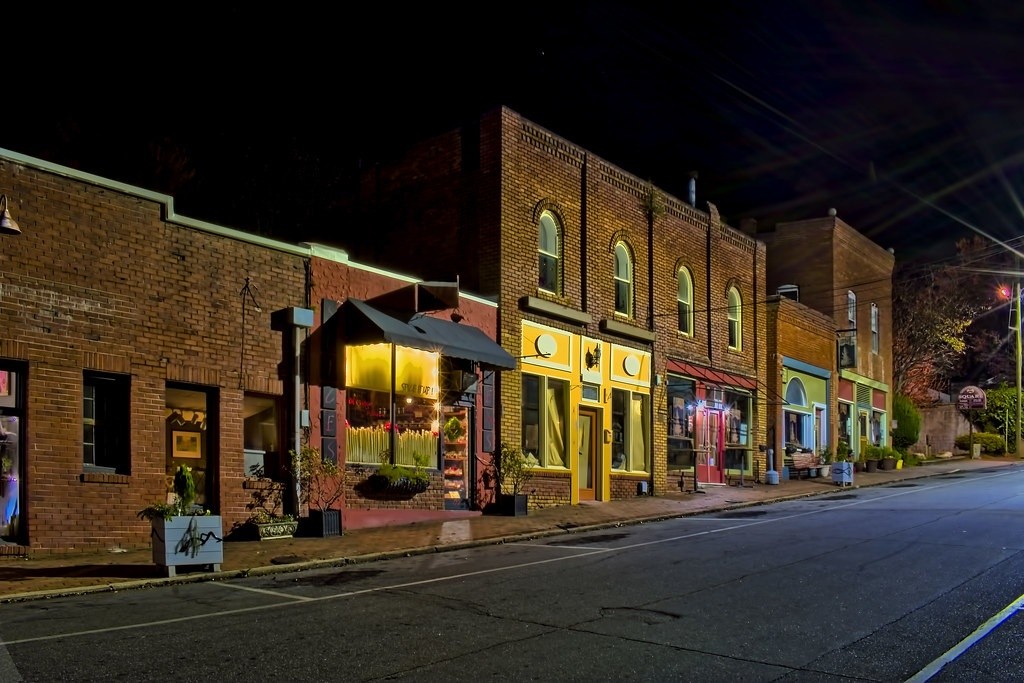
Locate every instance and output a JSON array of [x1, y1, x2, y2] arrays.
[[487, 441, 540, 517], [820, 436, 901, 486], [250, 511, 299, 542], [138, 463, 224, 576], [281, 446, 355, 538], [366, 449, 431, 494]]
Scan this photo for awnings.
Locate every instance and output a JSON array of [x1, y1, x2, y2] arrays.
[[348, 297, 516, 371]]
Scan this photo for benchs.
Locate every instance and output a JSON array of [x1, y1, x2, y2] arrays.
[[790, 452, 831, 480]]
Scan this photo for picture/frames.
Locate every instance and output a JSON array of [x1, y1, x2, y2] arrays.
[[171, 430, 202, 459]]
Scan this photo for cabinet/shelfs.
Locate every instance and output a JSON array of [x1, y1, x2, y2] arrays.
[[372, 410, 469, 492]]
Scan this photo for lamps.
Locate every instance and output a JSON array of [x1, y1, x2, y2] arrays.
[[0, 193, 22, 235], [588, 342, 601, 367]]
